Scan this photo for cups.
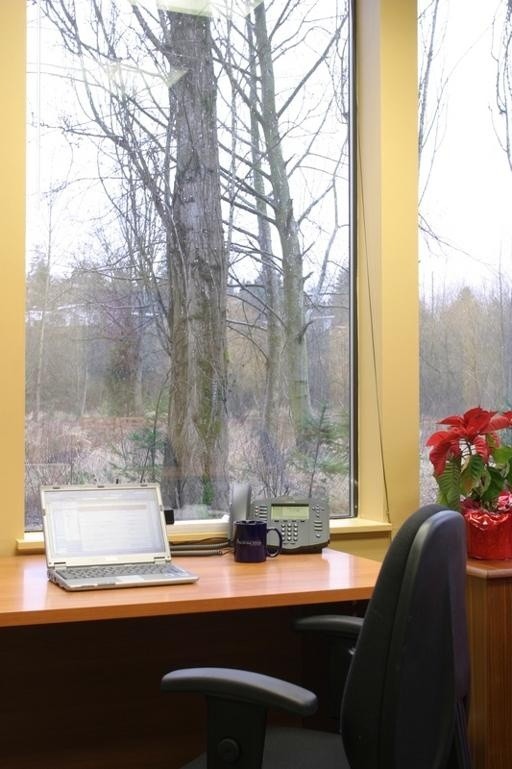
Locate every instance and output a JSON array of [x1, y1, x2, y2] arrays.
[[231, 519, 284, 565]]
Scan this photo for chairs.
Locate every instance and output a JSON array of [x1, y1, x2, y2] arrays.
[[160, 502, 473, 767]]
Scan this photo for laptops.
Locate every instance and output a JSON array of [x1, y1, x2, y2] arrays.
[[36, 481, 202, 592]]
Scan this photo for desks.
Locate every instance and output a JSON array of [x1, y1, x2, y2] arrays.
[[0, 538, 385, 768]]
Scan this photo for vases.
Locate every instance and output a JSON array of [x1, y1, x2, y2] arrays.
[[453, 490, 512, 561]]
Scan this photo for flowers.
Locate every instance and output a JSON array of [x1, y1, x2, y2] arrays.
[[424, 404, 512, 513]]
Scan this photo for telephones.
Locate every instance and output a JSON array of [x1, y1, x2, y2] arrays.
[[228, 483, 331, 553]]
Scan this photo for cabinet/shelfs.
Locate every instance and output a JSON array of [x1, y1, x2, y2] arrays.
[[453, 555, 510, 767]]
